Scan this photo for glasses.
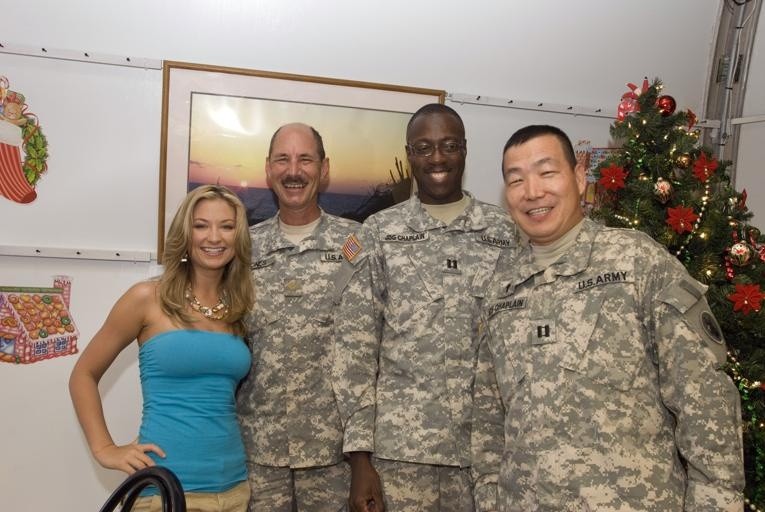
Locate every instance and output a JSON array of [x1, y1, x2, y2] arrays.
[[406, 140, 463, 158]]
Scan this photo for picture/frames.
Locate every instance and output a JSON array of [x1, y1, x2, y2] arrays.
[[155, 59, 449, 268]]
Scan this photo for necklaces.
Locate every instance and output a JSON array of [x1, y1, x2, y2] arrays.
[[185, 276, 232, 323]]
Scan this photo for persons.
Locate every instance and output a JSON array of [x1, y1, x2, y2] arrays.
[[234, 120, 364, 511], [333, 102, 525, 512], [68, 184, 256, 510], [467, 121, 750, 510]]
